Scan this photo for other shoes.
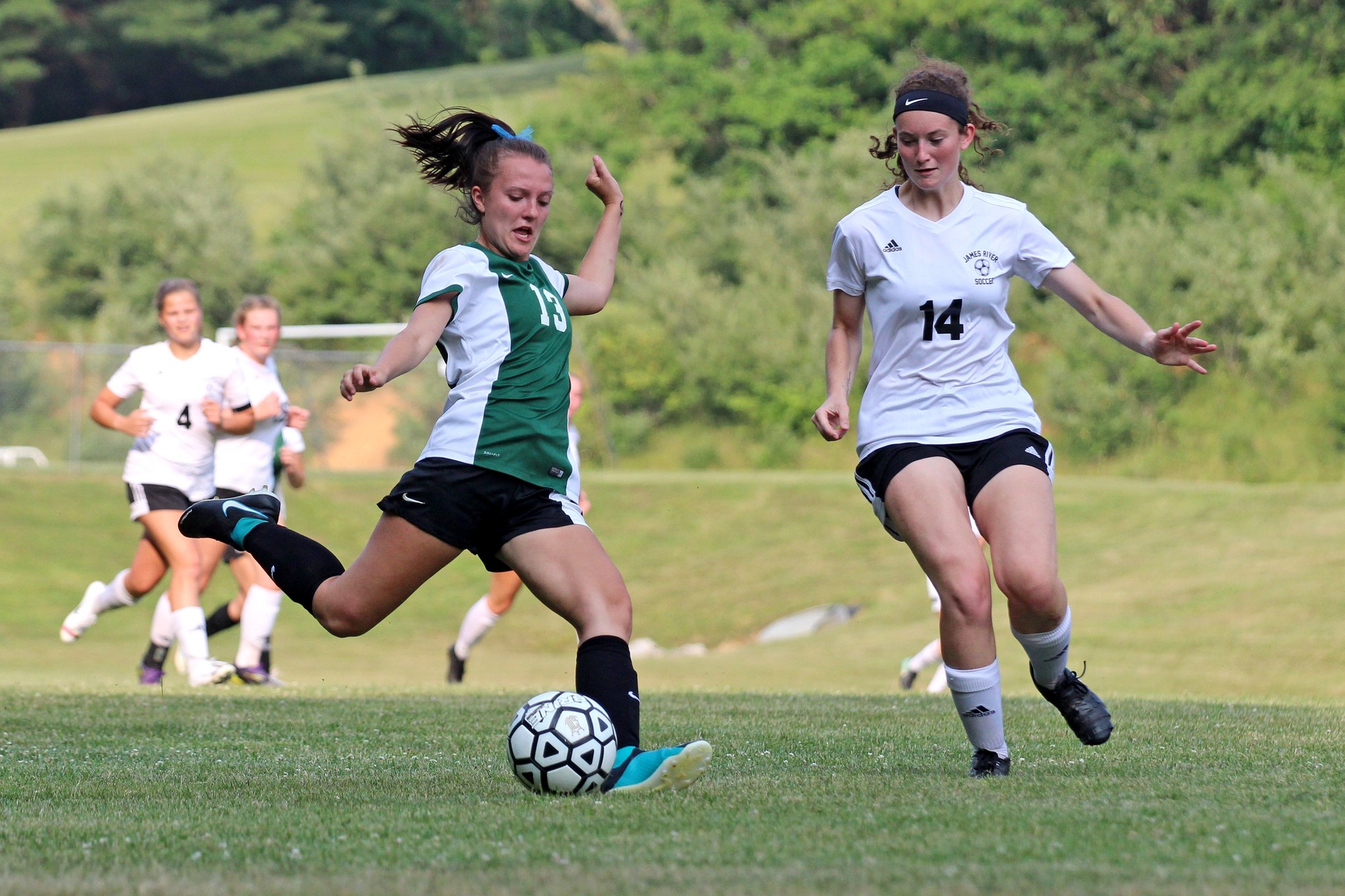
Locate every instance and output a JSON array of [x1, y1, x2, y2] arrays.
[[137, 659, 163, 686], [446, 641, 465, 684], [897, 657, 916, 693], [232, 662, 274, 687]]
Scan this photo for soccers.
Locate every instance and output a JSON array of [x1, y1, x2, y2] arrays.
[[506, 690, 618, 798]]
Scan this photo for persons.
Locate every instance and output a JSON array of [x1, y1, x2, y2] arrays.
[[178, 111, 713, 798], [136, 293, 307, 689], [898, 505, 988, 701], [811, 59, 1218, 779], [447, 372, 593, 687], [57, 276, 253, 687]]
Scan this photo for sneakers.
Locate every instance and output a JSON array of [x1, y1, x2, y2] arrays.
[[600, 738, 711, 798], [967, 747, 1013, 779], [58, 580, 107, 644], [186, 658, 235, 687], [1029, 657, 1114, 747], [177, 485, 281, 553]]
